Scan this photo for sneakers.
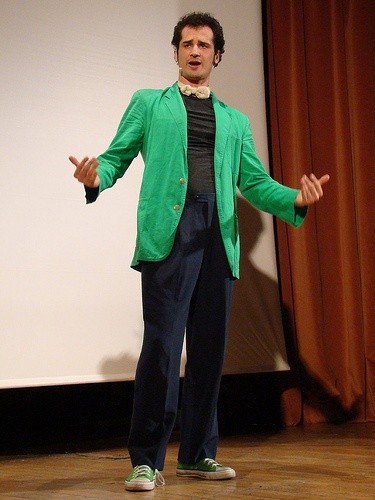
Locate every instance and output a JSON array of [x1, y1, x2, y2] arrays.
[[175, 458, 236, 480], [123, 464, 165, 491]]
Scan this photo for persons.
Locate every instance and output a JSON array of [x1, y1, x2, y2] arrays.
[[69, 12, 329, 490]]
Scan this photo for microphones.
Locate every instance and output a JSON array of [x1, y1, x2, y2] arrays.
[[179, 68, 182, 70]]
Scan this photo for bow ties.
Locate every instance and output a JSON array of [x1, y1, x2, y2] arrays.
[[176, 80, 213, 100]]
